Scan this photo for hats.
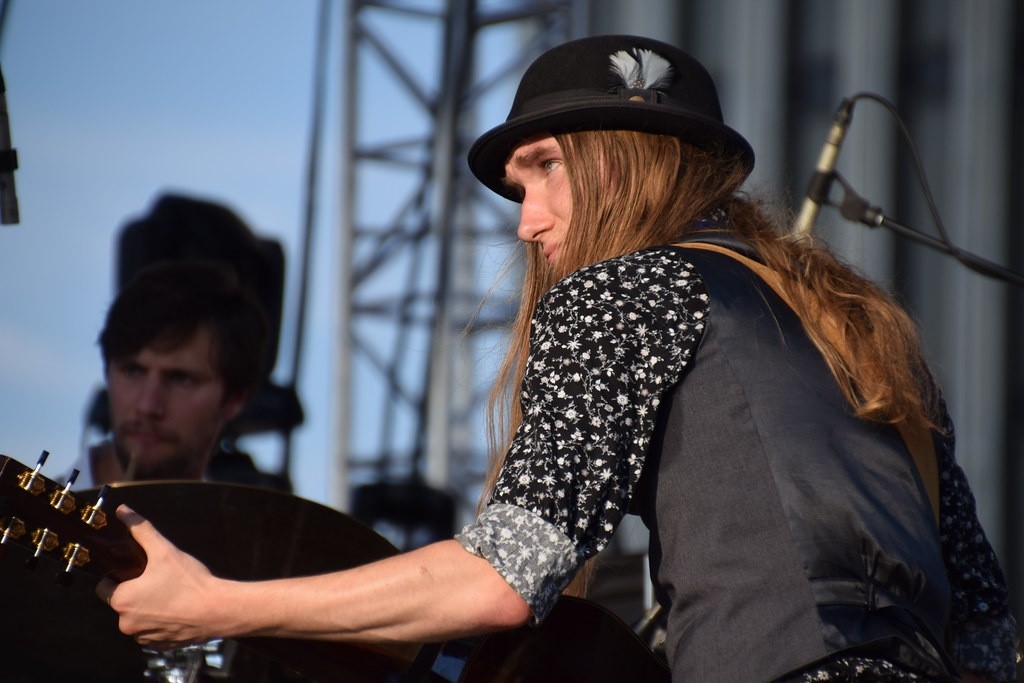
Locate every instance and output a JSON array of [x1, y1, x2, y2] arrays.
[[467, 33, 757, 205]]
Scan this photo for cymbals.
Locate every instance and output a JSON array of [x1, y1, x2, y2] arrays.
[[68, 478, 402, 585]]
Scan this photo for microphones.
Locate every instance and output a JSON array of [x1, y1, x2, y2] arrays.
[[791, 97, 855, 242]]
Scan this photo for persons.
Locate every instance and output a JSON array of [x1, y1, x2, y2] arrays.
[[51, 255, 262, 495], [98, 35, 1022, 681]]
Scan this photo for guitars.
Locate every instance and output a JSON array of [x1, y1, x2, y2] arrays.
[[0, 450, 672, 683]]
